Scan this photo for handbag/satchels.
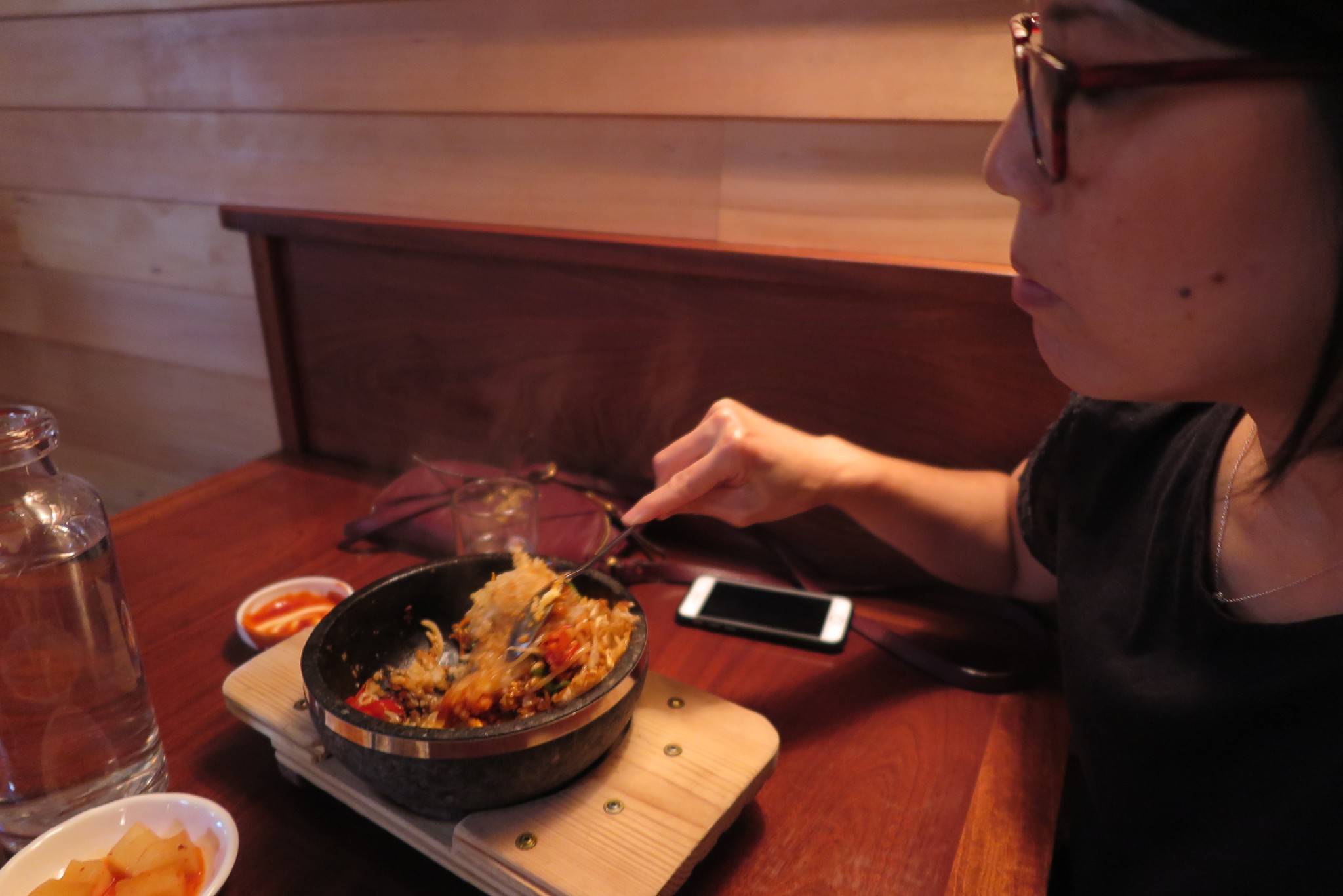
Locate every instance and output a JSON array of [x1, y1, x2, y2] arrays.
[[345, 457, 642, 569]]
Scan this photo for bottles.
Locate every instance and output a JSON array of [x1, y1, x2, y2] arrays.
[[0, 402, 172, 854]]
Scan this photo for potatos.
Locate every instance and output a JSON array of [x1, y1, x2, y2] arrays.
[[28, 822, 206, 896]]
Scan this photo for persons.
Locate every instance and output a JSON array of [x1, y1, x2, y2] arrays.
[[618, 0, 1343, 896]]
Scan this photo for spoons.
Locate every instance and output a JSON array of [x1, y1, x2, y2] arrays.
[[505, 526, 638, 661]]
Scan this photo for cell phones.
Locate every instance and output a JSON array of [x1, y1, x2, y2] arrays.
[[678, 575, 855, 651]]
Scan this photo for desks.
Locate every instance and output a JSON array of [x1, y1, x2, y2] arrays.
[[0, 450, 1069, 896]]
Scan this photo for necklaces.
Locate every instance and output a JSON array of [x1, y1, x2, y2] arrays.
[[1215, 429, 1343, 603]]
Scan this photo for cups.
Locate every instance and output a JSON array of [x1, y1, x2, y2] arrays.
[[453, 476, 539, 553]]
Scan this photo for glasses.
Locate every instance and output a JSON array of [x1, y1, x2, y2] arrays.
[[1010, 12, 1319, 183]]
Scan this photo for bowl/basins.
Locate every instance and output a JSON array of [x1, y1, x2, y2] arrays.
[[235, 576, 354, 651], [0, 792, 240, 896], [300, 551, 648, 820]]
